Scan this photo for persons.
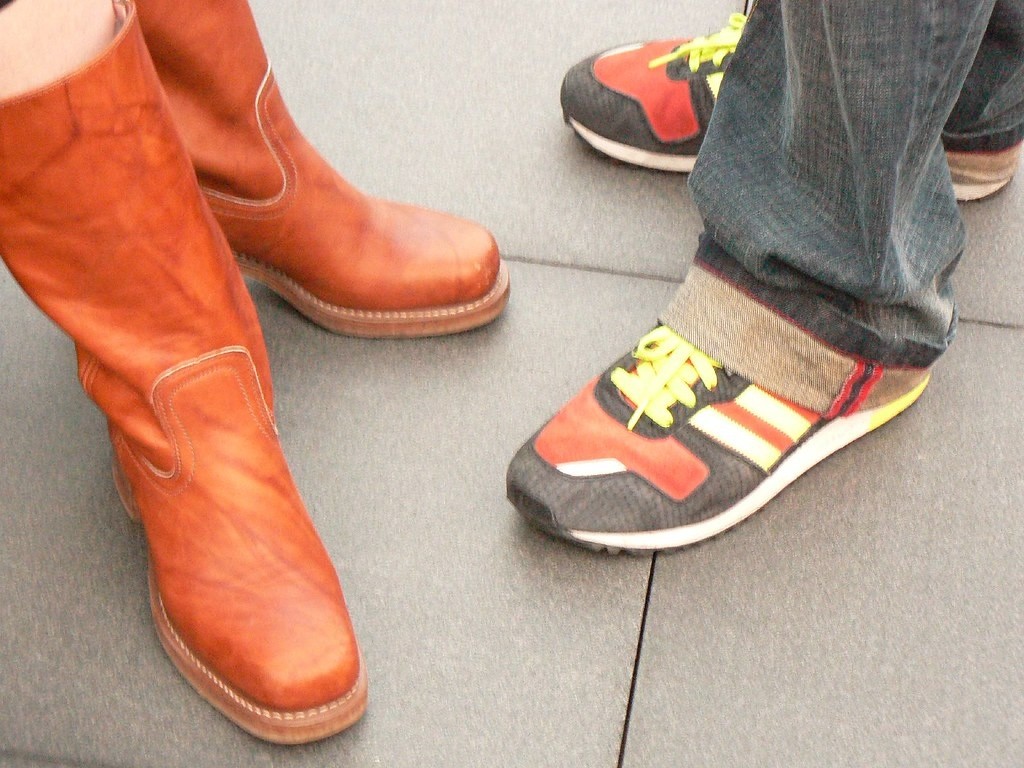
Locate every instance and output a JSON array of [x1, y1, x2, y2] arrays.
[[505, 0, 1024, 558], [0, 0, 511, 747]]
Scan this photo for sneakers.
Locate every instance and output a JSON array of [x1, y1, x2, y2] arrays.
[[505, 325, 933, 554], [561, 13, 1022, 202]]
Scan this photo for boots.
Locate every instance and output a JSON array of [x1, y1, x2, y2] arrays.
[[0, 0, 366, 740], [136, 0, 511, 335]]
